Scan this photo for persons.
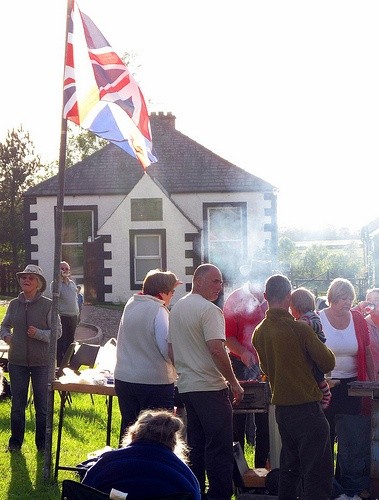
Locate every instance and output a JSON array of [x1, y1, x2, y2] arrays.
[[169, 263, 245, 500], [50, 261, 78, 373], [288, 287, 336, 410], [114, 272, 183, 427], [1, 265, 62, 453], [223, 259, 270, 494], [315, 278, 378, 500], [350, 290, 378, 499], [77, 286, 82, 323], [80, 411, 202, 499], [250, 273, 336, 500]]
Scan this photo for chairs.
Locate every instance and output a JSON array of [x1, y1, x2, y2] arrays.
[[26, 342, 100, 410]]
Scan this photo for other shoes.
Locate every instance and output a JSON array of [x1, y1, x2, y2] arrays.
[[8, 444, 22, 453], [37, 447, 45, 453]]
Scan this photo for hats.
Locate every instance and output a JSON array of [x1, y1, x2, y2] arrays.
[[240, 259, 273, 282], [16, 264, 47, 292]]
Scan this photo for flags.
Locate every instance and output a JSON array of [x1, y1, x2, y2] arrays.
[[63, 0, 159, 170]]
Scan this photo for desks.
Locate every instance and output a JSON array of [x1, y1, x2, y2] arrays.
[[51, 380, 119, 479]]
